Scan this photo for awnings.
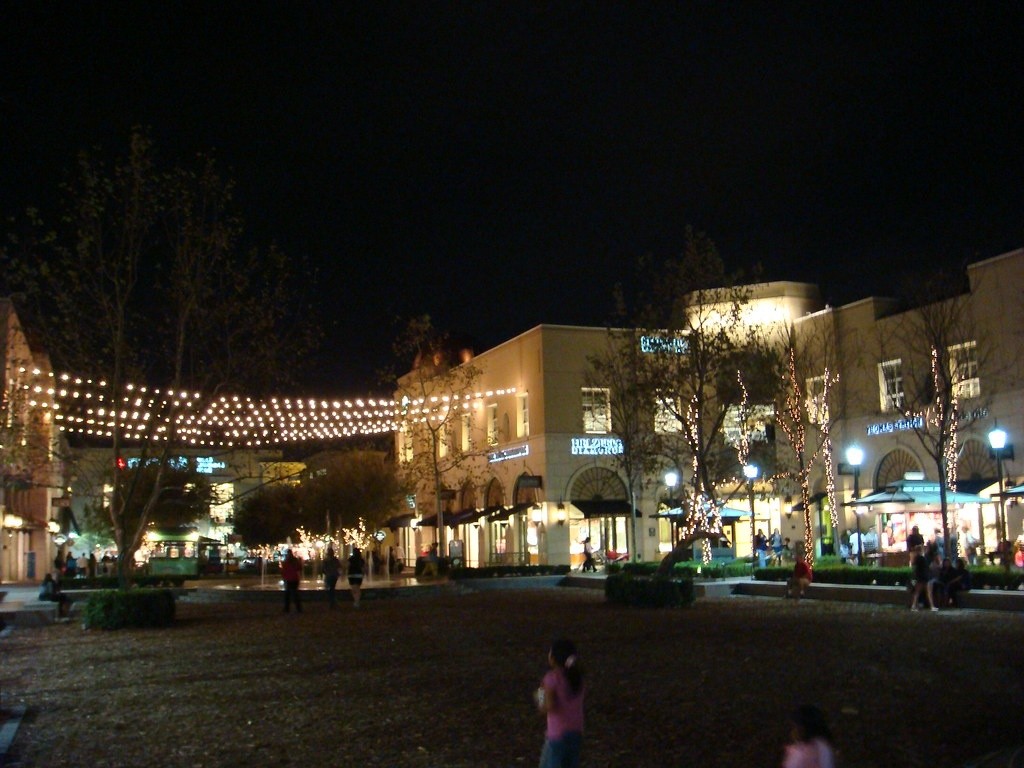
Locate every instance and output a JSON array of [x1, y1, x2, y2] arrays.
[[455, 502, 535, 522], [568, 500, 642, 519], [416, 508, 478, 529], [662, 495, 725, 510]]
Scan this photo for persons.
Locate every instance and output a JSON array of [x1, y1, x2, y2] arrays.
[[906, 520, 980, 612], [200, 544, 208, 578], [783, 537, 791, 552], [389, 542, 405, 575], [756, 528, 768, 569], [38, 573, 69, 617], [421, 541, 438, 556], [101, 555, 117, 574], [347, 548, 365, 607], [996, 529, 1024, 569], [281, 549, 304, 614], [582, 537, 598, 573], [533, 637, 589, 768], [781, 704, 834, 768], [54, 549, 97, 578], [839, 522, 894, 566], [321, 547, 342, 600], [784, 548, 813, 600], [768, 528, 783, 567]]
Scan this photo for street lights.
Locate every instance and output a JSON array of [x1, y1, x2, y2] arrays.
[[987, 416, 1006, 541], [845, 441, 865, 567], [743, 463, 759, 554], [664, 470, 678, 552]]
